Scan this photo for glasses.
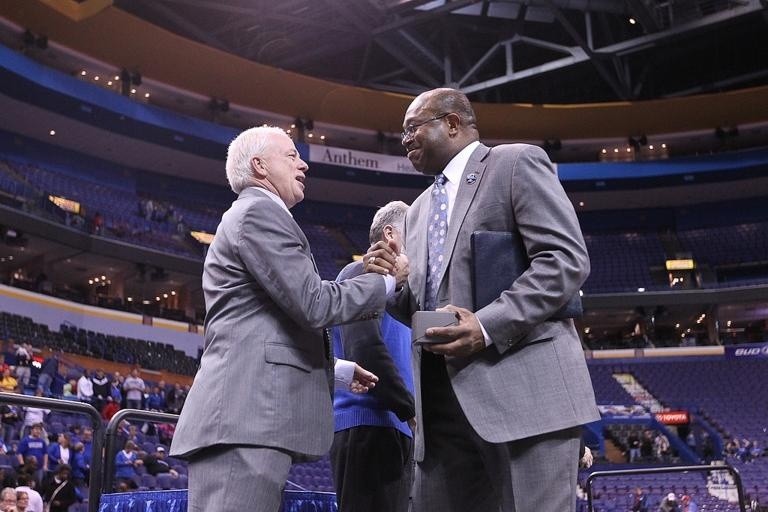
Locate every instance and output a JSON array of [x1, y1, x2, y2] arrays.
[[400, 112, 452, 141]]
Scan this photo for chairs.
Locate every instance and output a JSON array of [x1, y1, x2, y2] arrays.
[[279, 457, 338, 510], [575, 357, 768, 511], [1, 312, 202, 511]]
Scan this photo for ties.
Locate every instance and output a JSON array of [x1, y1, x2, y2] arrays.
[[424, 173, 450, 311]]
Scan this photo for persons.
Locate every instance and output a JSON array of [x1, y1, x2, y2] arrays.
[[0, 336, 190, 512], [168, 126, 412, 512], [328, 200, 432, 512], [573, 410, 768, 512], [362, 88, 602, 512]]
